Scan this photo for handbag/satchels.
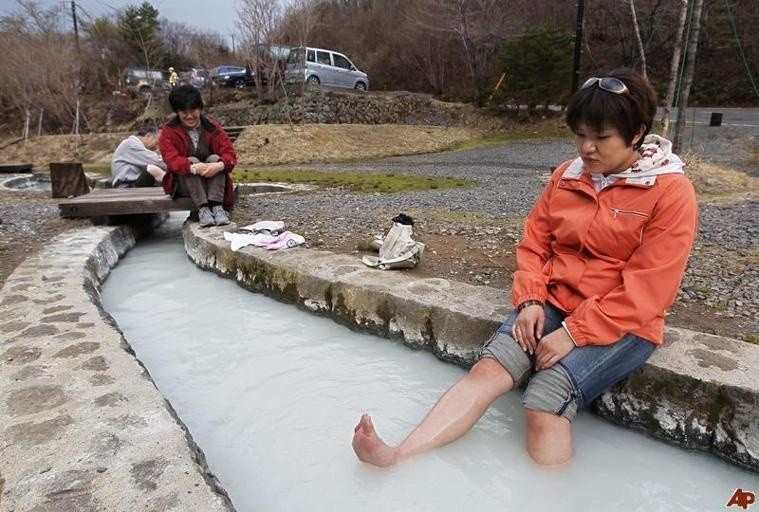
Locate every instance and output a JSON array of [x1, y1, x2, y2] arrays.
[[359, 220, 425, 271], [239, 216, 287, 237]]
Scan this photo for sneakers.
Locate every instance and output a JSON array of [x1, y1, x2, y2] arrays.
[[198, 205, 215, 228], [212, 204, 231, 226]]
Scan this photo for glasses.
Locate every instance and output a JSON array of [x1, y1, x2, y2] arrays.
[[582, 75, 629, 95]]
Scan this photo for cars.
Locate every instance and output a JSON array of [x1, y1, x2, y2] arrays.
[[120, 62, 277, 97]]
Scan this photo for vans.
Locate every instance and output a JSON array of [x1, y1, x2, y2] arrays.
[[286, 45, 371, 93]]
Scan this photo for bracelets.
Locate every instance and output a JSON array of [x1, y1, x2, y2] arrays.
[[517, 300, 544, 314]]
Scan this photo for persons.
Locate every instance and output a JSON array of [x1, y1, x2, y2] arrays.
[[168, 67, 177, 89], [353, 67, 697, 467], [159, 84, 237, 227], [111, 126, 167, 188]]
